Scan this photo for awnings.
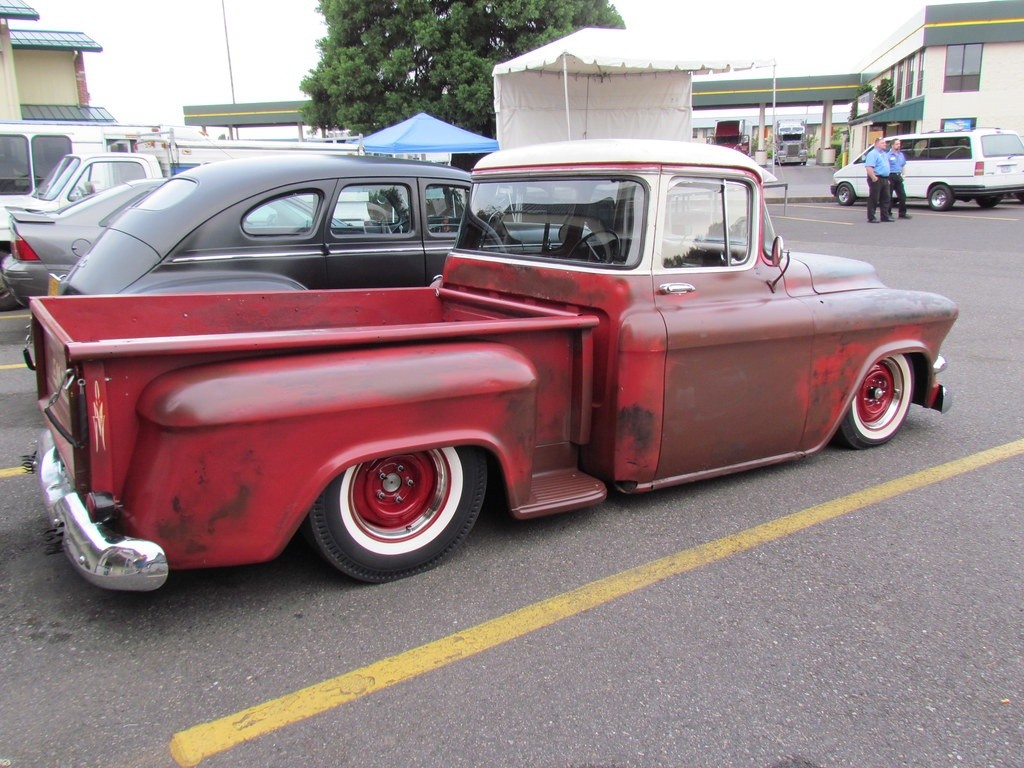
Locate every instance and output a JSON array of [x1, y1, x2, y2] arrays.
[[850, 95, 924, 125]]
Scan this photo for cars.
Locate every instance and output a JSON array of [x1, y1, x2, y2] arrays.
[[0, 123, 354, 315], [41, 147, 607, 298]]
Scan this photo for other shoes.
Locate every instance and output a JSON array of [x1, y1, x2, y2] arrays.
[[900, 214, 912, 219], [881, 217, 895, 221], [868, 218, 879, 223]]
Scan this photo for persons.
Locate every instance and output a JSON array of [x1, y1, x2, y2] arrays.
[[887, 139, 912, 219], [865, 138, 895, 223]]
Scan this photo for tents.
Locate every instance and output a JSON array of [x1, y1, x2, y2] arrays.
[[355, 110, 499, 168], [495, 27, 776, 177]]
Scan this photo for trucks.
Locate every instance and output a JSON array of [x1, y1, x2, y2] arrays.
[[774, 120, 808, 166]]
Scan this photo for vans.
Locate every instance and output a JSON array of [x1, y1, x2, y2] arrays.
[[829, 127, 1024, 211]]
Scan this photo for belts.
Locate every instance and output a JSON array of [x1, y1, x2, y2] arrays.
[[890, 173, 900, 176]]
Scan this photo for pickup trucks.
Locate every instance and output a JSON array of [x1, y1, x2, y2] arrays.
[[17, 135, 964, 593]]
